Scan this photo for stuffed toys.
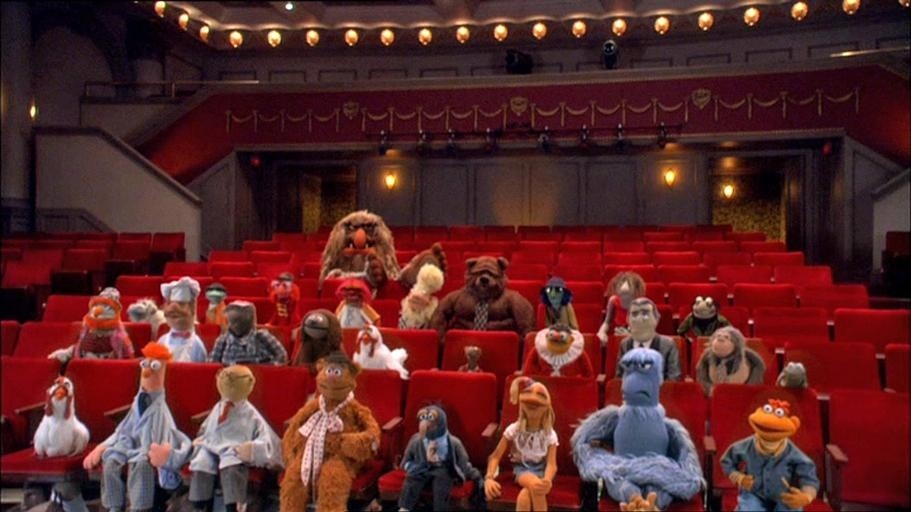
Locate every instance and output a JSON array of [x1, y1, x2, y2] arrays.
[[279, 353, 381, 512], [719, 397, 821, 512], [570, 347, 704, 510], [317, 210, 447, 289], [47, 256, 809, 398], [33, 376, 90, 458], [47, 256, 808, 399], [397, 405, 484, 512]]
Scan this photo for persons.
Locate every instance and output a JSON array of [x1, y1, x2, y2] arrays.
[[195, 364, 282, 510], [82, 341, 192, 512], [484, 376, 560, 511]]
